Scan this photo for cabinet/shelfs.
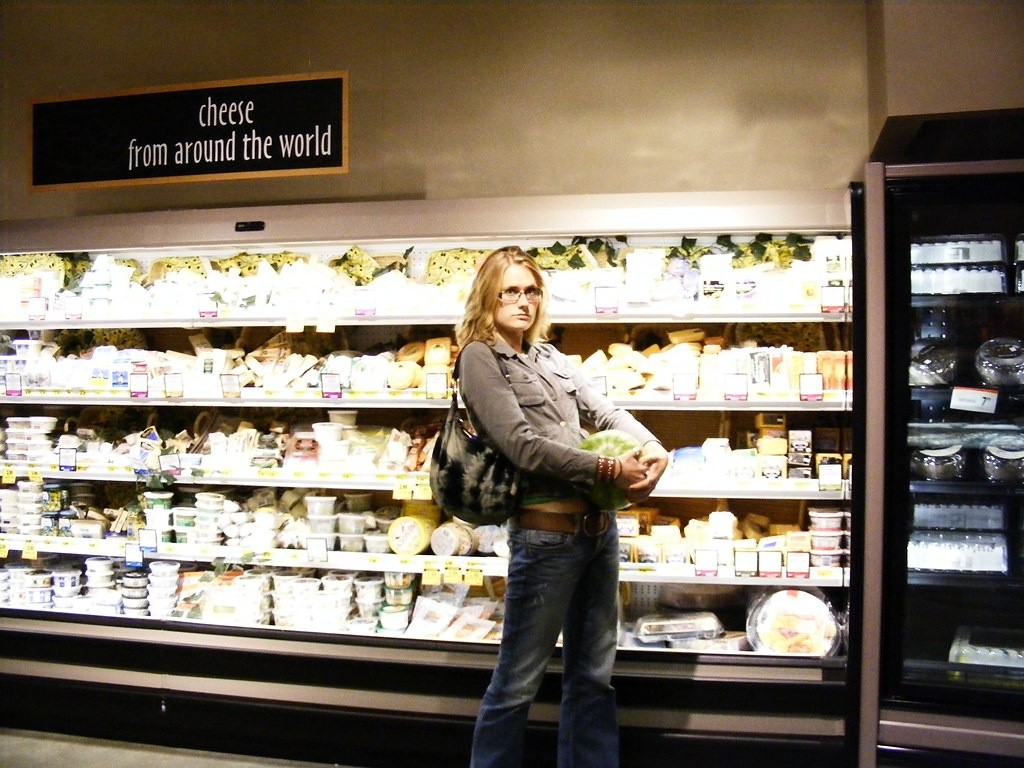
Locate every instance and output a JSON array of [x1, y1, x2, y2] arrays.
[[0, 181, 866, 768]]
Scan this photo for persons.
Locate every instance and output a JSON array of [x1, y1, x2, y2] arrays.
[[458, 245, 667, 768]]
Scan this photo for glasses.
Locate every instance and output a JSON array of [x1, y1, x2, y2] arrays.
[[498, 286, 544, 301]]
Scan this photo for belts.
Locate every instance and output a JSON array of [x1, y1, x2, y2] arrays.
[[507, 506, 619, 537]]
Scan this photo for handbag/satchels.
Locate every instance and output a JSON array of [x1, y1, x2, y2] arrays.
[[429, 340, 522, 526]]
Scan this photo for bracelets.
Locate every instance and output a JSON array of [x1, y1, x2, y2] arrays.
[[596, 457, 614, 481], [615, 458, 622, 480]]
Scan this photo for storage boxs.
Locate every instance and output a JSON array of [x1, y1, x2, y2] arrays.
[[0, 236, 853, 656]]
